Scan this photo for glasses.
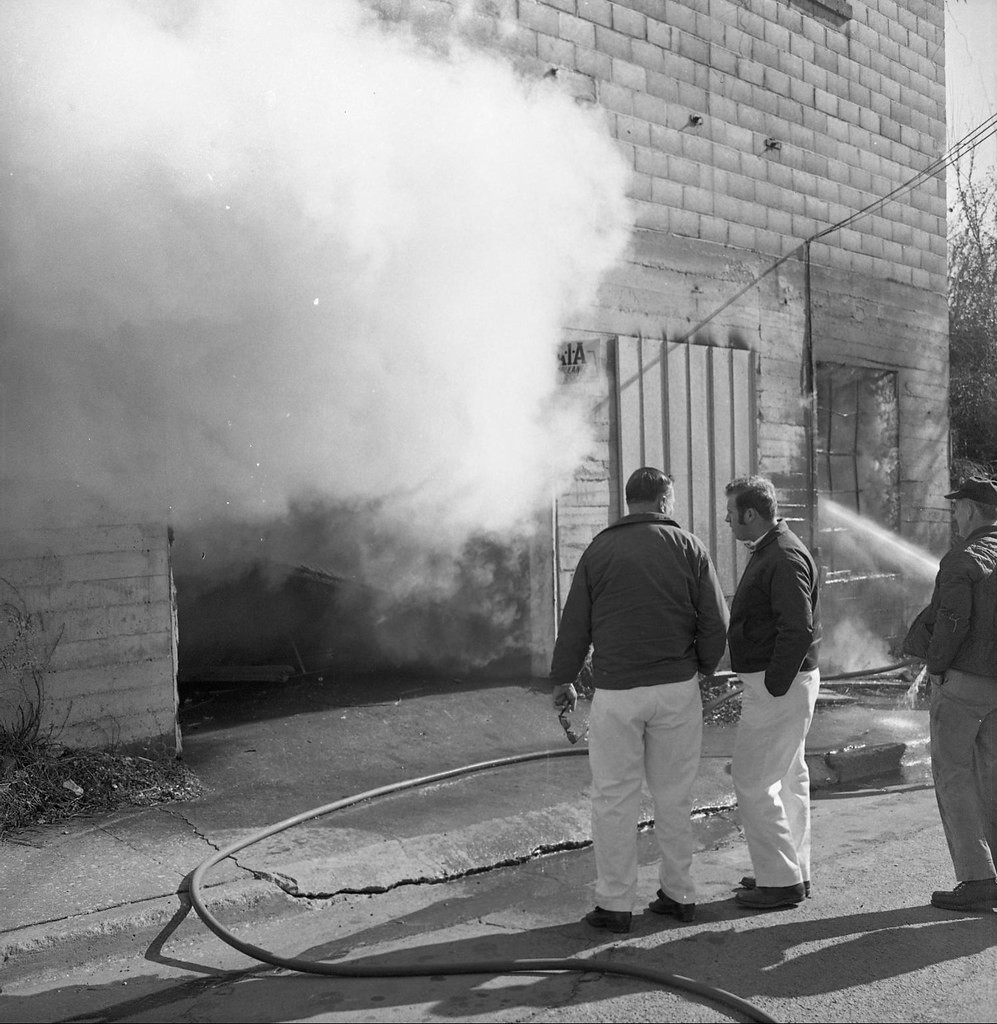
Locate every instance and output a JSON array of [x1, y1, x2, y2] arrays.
[[951, 499, 957, 510], [558, 695, 590, 746]]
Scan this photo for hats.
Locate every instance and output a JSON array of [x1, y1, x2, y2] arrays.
[[944, 475, 997, 507]]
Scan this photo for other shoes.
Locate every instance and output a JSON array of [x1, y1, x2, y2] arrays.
[[649, 889, 696, 924], [932, 879, 997, 918], [587, 906, 633, 935], [733, 877, 811, 909]]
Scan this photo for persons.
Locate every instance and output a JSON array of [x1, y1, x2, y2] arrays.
[[903, 476, 997, 911], [726, 476, 822, 909], [550, 467, 731, 933]]
